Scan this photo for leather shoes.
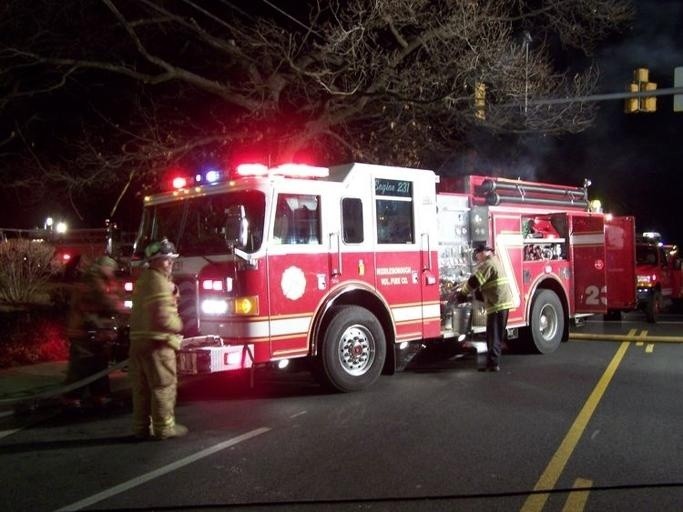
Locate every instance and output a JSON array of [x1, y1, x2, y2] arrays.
[[478, 365, 500, 372]]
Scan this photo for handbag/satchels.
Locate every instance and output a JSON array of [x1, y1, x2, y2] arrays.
[[84, 326, 121, 348]]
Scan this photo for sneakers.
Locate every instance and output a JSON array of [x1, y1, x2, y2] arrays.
[[154, 425, 188, 441], [136, 424, 155, 442]]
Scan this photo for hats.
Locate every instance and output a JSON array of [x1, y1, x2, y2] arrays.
[[472, 245, 494, 261], [144, 238, 180, 263]]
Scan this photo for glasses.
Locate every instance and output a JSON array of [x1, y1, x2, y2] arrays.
[[160, 241, 177, 255]]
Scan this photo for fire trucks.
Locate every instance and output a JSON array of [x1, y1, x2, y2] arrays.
[[603, 230, 682, 322], [114, 160, 642, 393]]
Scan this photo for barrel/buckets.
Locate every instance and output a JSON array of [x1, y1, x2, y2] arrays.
[[452, 302, 473, 335]]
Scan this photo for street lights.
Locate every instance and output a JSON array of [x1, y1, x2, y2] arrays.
[[43, 216, 52, 230]]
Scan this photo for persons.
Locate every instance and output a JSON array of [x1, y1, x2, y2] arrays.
[[122, 237, 189, 440], [454, 244, 520, 373], [61, 255, 121, 410]]
